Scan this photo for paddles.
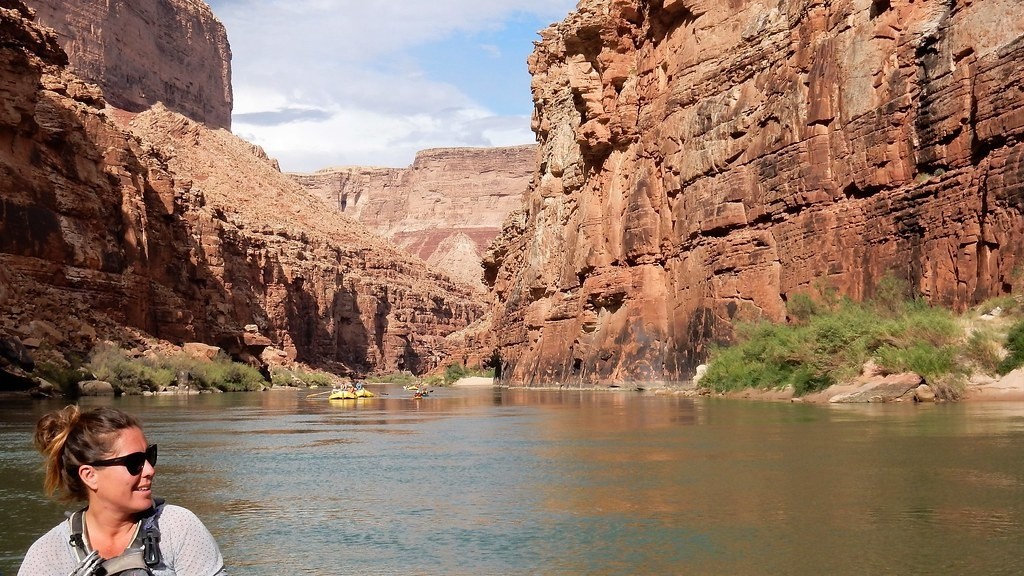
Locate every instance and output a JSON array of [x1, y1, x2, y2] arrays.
[[307, 390, 331, 398]]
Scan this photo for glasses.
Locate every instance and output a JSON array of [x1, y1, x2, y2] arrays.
[[87, 444, 157, 476]]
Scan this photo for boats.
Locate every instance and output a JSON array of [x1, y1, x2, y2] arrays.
[[404, 384, 430, 399], [328, 388, 358, 400], [356, 387, 375, 398]]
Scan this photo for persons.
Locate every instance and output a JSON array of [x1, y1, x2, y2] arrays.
[[15, 405, 228, 576], [415, 388, 428, 398], [336, 379, 362, 393]]
